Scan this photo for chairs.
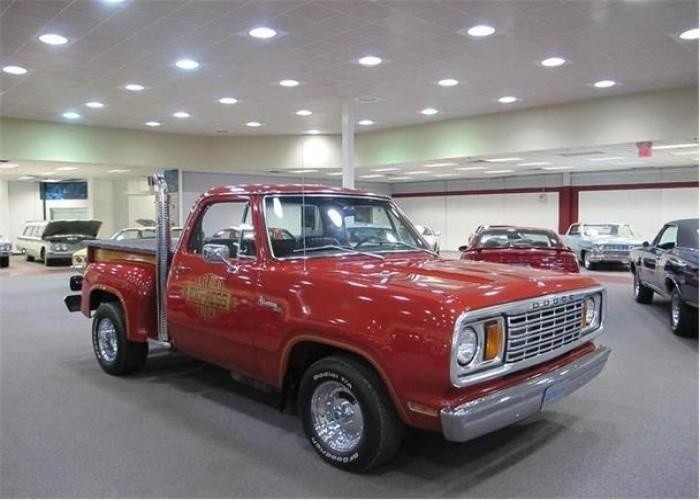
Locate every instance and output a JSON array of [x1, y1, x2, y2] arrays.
[[203, 226, 388, 263]]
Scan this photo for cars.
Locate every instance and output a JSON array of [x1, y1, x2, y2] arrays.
[[16, 220, 104, 266], [71, 228, 185, 274], [459, 224, 580, 275], [413, 225, 442, 254], [629, 218, 699, 336], [343, 224, 398, 250], [208, 224, 296, 243]]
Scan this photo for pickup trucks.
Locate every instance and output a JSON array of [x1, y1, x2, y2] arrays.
[[64, 184, 611, 470], [558, 222, 638, 271]]
